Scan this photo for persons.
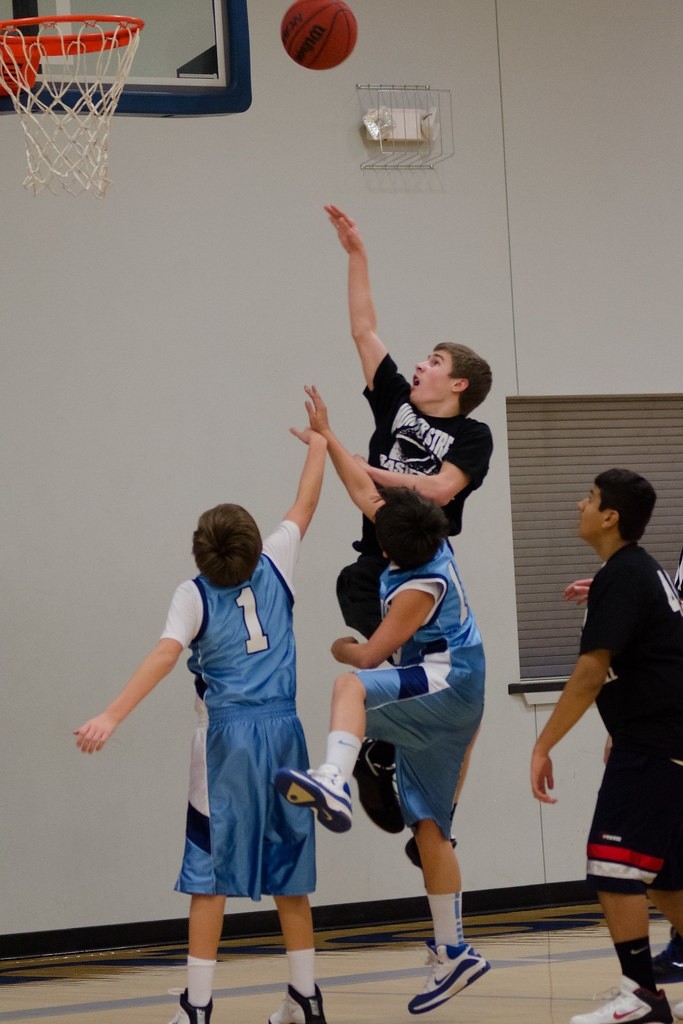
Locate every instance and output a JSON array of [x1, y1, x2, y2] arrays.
[[273, 387, 491, 1013], [528, 468, 683, 1024], [324, 202, 495, 866], [73, 426, 328, 1024]]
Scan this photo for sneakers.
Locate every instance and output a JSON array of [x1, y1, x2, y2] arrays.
[[168, 988, 213, 1024], [267, 982, 327, 1024], [570, 975, 673, 1024], [407, 938, 490, 1015], [403, 835, 428, 869], [651, 926, 683, 984], [354, 739, 404, 835], [274, 763, 353, 835]]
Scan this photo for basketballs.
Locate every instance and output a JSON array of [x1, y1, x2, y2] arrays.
[[280, 0, 360, 72]]
